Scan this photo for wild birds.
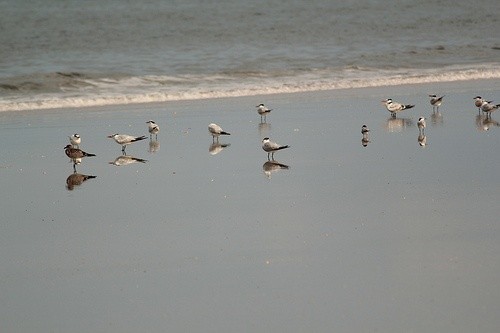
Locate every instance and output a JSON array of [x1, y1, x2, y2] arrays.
[[262, 157, 290, 179], [417, 116, 427, 131], [261, 137, 290, 157], [381, 98, 415, 119], [418, 132, 427, 148], [472, 96, 500, 121], [67, 133, 81, 148], [207, 140, 231, 155], [256, 104, 273, 119], [107, 133, 148, 151], [361, 124, 371, 136], [65, 169, 98, 192], [207, 123, 231, 140], [257, 119, 271, 136], [429, 94, 444, 114], [145, 119, 160, 138], [64, 144, 97, 169], [107, 151, 148, 166], [71, 158, 81, 165], [146, 138, 161, 153], [361, 137, 371, 147]]
